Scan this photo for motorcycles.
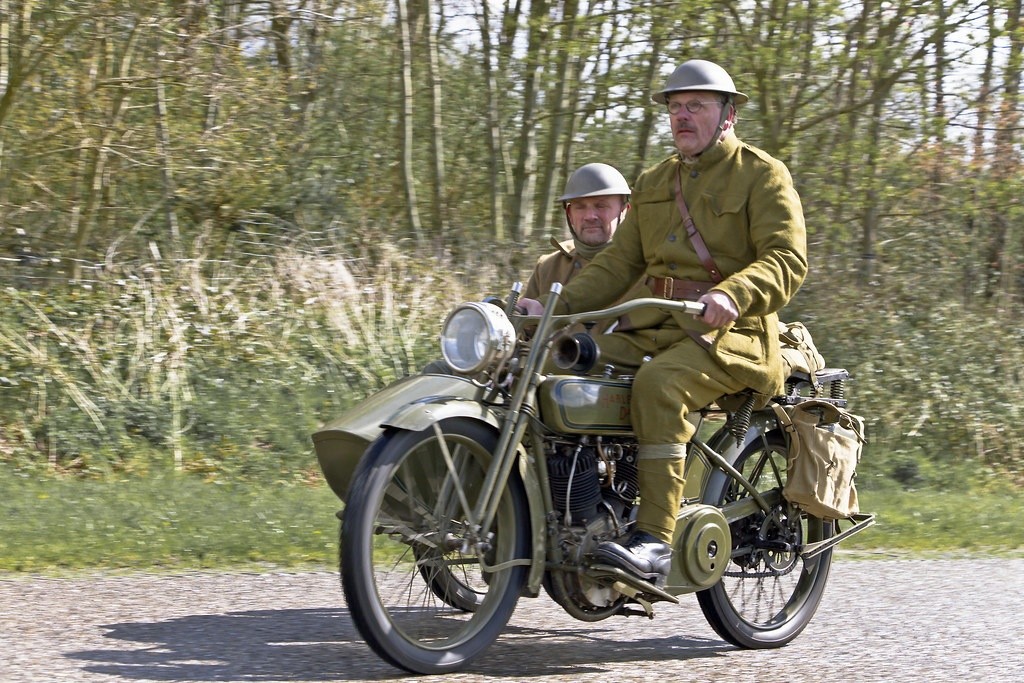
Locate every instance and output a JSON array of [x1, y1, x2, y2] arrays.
[[311, 278, 877, 676]]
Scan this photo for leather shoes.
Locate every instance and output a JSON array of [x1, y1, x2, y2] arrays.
[[594, 531, 671, 590]]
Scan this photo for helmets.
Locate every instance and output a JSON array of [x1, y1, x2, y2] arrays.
[[558, 163, 632, 200], [652, 60, 749, 104]]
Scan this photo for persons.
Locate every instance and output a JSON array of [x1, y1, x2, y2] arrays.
[[508, 58, 810, 580], [525, 163, 631, 300]]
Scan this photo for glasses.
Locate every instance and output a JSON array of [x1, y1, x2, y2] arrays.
[[667, 99, 720, 114]]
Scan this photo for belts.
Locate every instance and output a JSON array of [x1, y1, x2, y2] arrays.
[[646, 278, 718, 300]]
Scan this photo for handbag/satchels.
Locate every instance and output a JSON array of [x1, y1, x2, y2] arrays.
[[779, 319, 824, 382], [768, 399, 866, 521]]
